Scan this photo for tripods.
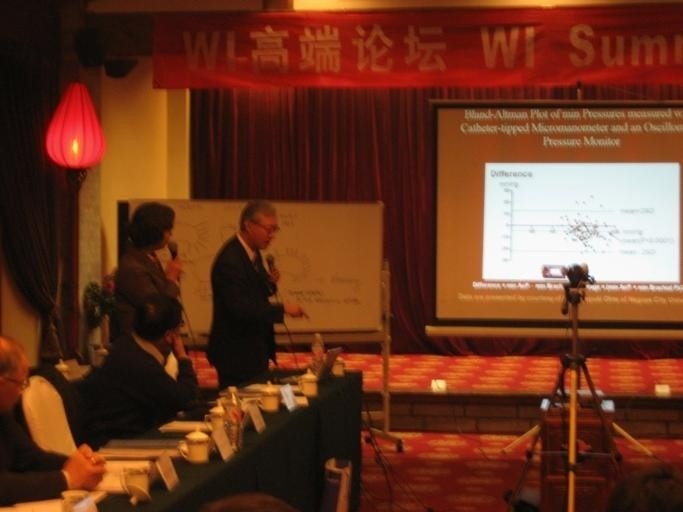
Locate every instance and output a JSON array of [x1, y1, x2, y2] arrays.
[[501, 301, 634, 512]]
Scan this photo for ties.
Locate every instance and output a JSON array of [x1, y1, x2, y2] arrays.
[[255, 247, 267, 276]]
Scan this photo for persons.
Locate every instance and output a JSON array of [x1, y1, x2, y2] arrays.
[[209, 200, 310, 391], [0, 336, 108, 507], [80, 294, 204, 440], [108, 201, 184, 354]]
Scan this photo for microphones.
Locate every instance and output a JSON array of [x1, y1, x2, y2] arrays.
[[265, 255, 276, 276], [168, 242, 179, 264]]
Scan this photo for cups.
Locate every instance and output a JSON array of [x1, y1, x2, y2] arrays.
[[178, 428, 208, 462], [121, 460, 149, 495], [204, 401, 228, 431], [62, 490, 88, 509], [254, 381, 279, 411], [297, 371, 317, 397]]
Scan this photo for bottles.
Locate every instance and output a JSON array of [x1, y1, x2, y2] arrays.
[[222, 382, 243, 448], [311, 332, 324, 366]]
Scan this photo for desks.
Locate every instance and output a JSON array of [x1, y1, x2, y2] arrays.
[[82, 366, 362, 511]]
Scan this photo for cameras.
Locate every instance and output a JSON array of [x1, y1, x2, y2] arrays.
[[542, 263, 589, 315]]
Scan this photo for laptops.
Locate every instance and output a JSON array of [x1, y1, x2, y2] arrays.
[[275, 344, 343, 385]]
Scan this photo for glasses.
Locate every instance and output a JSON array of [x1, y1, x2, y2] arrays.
[[2, 376, 30, 391], [251, 220, 280, 232]]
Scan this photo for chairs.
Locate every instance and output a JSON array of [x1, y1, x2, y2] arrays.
[[22, 375, 77, 457]]
